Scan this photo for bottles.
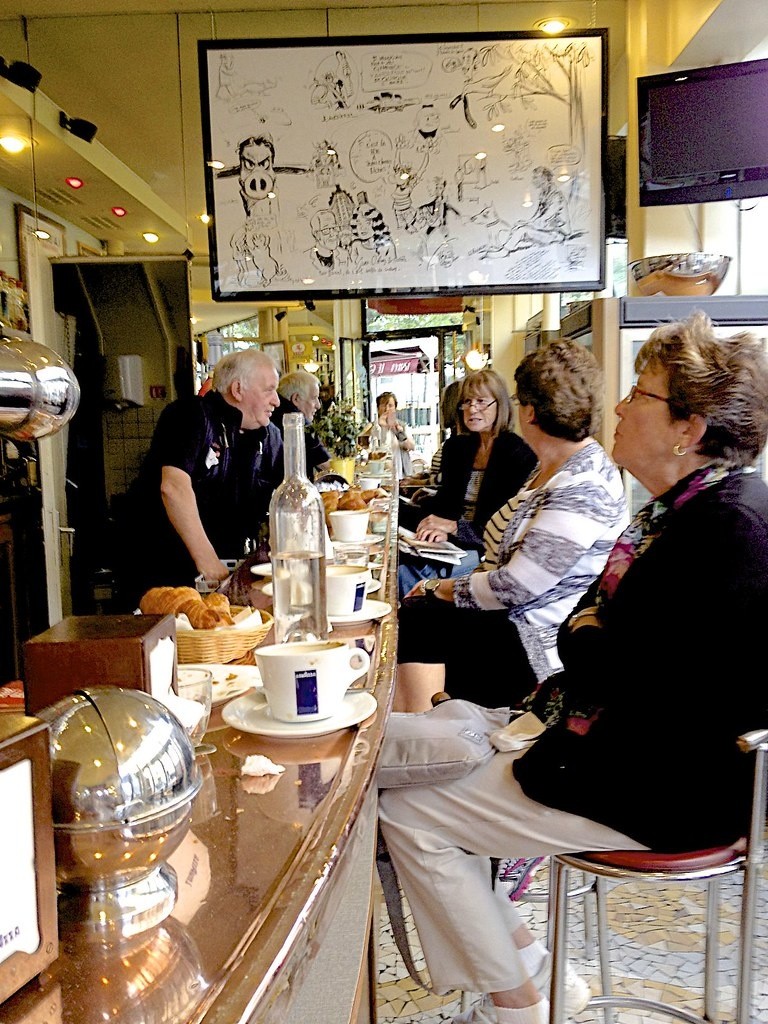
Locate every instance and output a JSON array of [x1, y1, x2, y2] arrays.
[[268, 412, 329, 645], [370, 414, 382, 446]]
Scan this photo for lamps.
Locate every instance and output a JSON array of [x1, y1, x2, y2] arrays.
[[274, 307, 289, 322], [59, 110, 99, 144], [463, 304, 475, 313], [0, 54, 43, 94]]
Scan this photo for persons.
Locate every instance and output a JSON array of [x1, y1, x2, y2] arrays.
[[378, 308, 768, 1024], [113, 349, 284, 614], [392, 338, 630, 900], [269, 370, 335, 483], [368, 370, 538, 600]]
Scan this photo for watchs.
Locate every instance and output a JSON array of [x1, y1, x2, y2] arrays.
[[568, 611, 596, 632], [424, 578, 441, 596]]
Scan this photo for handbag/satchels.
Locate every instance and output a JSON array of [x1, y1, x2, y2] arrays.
[[377, 700, 511, 789]]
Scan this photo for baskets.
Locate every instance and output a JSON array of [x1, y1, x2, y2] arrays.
[[178, 607, 274, 665]]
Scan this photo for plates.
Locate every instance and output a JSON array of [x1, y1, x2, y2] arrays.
[[328, 599, 392, 627], [360, 472, 393, 478], [176, 663, 251, 710], [251, 563, 382, 597], [220, 690, 378, 740], [331, 534, 385, 547]]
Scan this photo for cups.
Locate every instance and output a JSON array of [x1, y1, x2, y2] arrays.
[[177, 667, 213, 750], [332, 543, 370, 568], [253, 638, 370, 723]]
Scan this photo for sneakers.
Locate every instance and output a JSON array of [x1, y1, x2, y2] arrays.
[[452, 953, 592, 1024]]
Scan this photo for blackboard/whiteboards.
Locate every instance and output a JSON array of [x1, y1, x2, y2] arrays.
[[196, 26, 610, 303]]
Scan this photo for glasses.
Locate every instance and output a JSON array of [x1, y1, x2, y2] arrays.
[[457, 397, 499, 412], [511, 391, 535, 406], [626, 385, 672, 408]]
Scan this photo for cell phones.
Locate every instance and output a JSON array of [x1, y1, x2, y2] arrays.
[[387, 413, 396, 426]]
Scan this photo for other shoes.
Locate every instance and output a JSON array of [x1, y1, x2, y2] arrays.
[[500, 855, 550, 900]]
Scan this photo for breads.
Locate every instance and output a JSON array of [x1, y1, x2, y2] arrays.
[[321, 488, 387, 538], [140, 586, 238, 629]]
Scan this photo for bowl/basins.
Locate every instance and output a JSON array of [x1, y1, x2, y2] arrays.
[[369, 462, 385, 474], [359, 478, 382, 491], [629, 253, 732, 297], [326, 565, 371, 617], [328, 510, 370, 542]]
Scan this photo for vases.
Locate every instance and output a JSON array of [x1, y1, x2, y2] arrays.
[[333, 456, 355, 485]]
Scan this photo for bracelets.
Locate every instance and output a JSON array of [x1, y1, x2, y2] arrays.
[[420, 579, 429, 594]]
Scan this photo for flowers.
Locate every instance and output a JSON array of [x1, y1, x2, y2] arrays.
[[306, 399, 368, 459]]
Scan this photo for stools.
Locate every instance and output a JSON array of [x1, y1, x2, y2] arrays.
[[488, 729, 768, 1024]]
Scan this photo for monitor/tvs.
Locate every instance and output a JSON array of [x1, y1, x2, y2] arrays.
[[637, 59, 768, 207]]
